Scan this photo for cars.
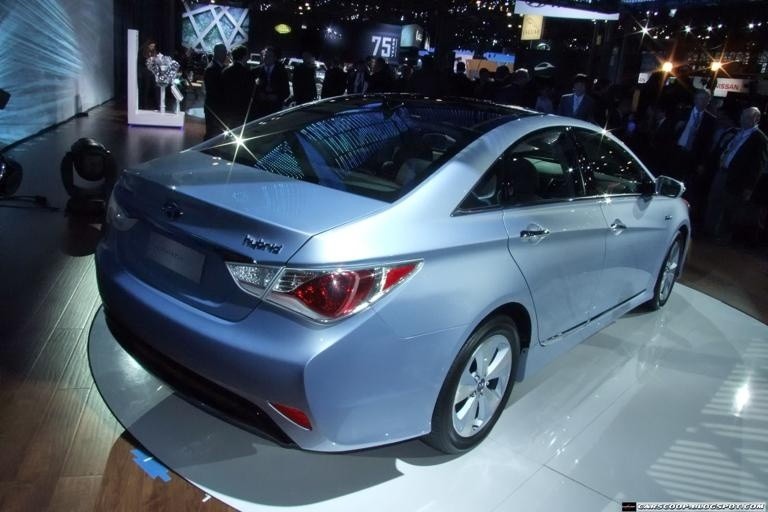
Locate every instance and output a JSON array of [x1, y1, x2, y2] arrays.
[[93, 87, 695, 456]]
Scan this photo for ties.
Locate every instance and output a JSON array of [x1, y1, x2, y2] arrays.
[[685, 112, 699, 150], [719, 131, 743, 169]]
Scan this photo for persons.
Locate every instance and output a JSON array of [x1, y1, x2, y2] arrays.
[[137, 38, 767, 245]]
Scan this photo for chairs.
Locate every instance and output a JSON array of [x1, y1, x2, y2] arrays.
[[392, 126, 433, 170], [497, 156, 543, 204]]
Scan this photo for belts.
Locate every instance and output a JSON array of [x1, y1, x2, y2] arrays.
[[679, 146, 687, 152]]
